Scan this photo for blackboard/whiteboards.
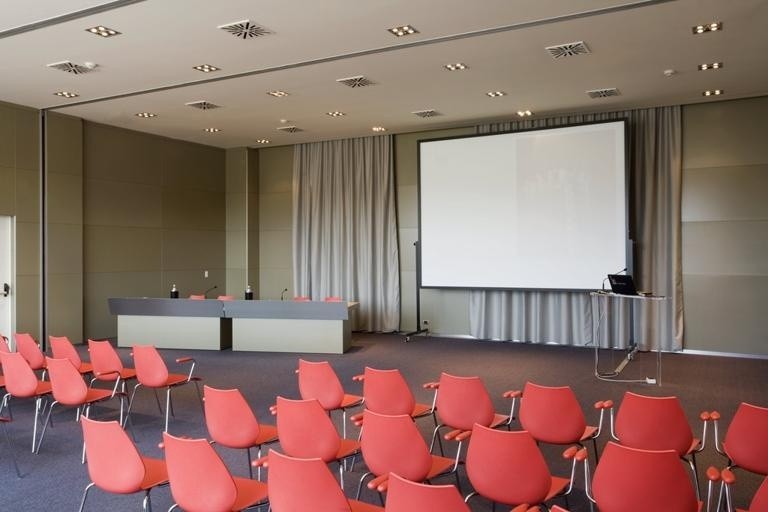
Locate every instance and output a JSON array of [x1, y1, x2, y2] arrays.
[[417, 117, 629, 293]]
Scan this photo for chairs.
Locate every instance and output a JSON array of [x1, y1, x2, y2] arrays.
[[0, 330, 216, 478]]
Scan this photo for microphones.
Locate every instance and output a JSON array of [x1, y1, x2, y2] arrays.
[[205, 285, 217, 298], [602, 267, 628, 290], [280, 288, 288, 300]]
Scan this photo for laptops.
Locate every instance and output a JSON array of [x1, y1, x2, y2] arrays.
[[608, 274, 638, 295]]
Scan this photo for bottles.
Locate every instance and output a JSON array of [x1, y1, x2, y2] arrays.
[[171, 284, 178, 299], [244, 285, 253, 300]]
